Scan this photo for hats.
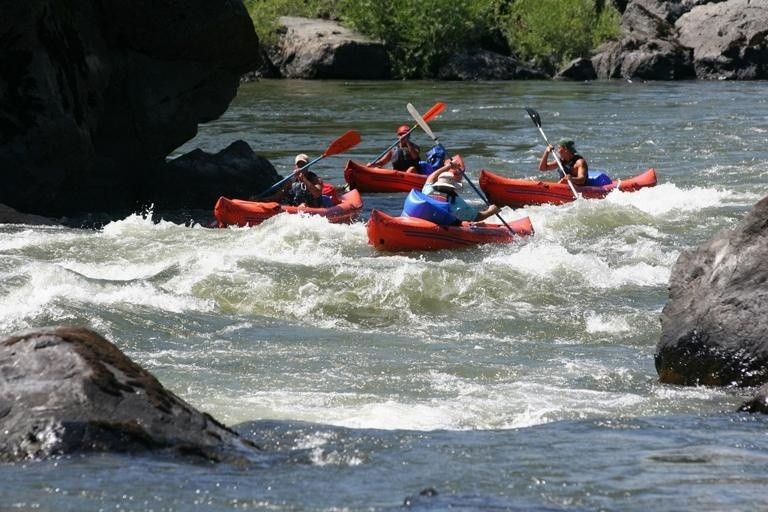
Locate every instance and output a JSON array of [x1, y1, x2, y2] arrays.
[[431, 171, 463, 190], [295, 153, 310, 165], [558, 137, 578, 153], [397, 124, 410, 134]]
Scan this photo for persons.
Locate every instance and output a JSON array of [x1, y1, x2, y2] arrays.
[[250, 153, 323, 208], [366, 125, 421, 174], [421, 161, 499, 226], [538, 137, 588, 186]]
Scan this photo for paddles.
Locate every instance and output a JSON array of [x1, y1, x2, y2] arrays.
[[367, 102, 446, 167], [525, 105, 584, 202], [245, 130, 362, 200], [406, 102, 526, 246]]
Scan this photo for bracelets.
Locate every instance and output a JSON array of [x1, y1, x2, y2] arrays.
[[448, 162, 452, 166], [571, 177, 574, 183]]
